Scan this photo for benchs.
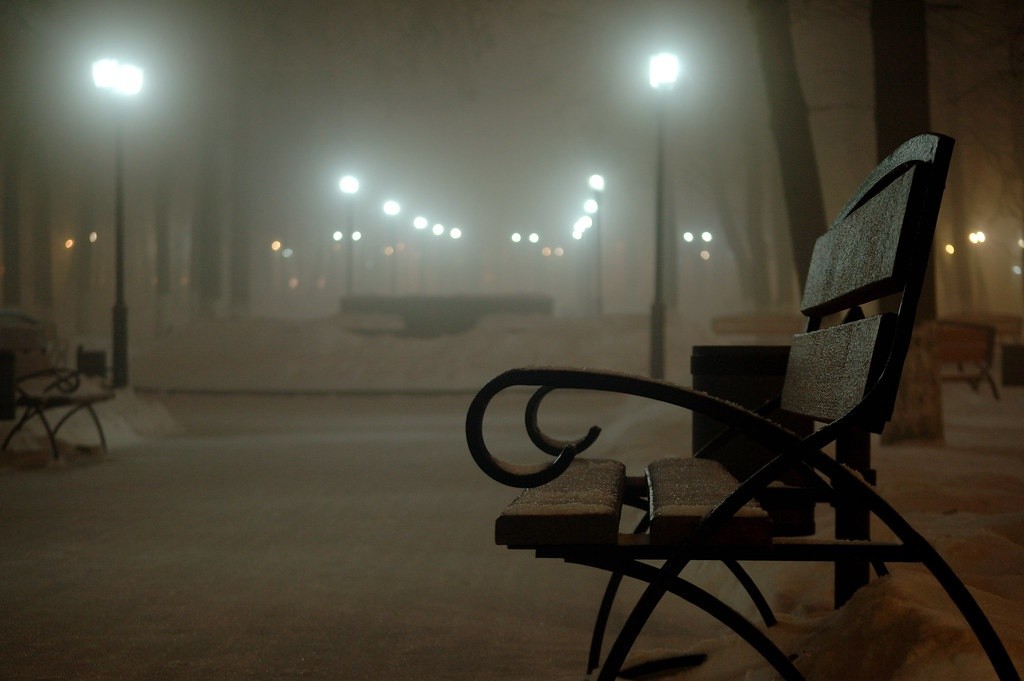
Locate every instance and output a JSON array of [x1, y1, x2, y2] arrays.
[[0, 328, 116, 462], [465, 133, 1021, 681]]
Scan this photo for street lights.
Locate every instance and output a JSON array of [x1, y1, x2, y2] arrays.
[[646, 51, 680, 380], [337, 176, 359, 296], [93, 59, 145, 389], [588, 173, 605, 314]]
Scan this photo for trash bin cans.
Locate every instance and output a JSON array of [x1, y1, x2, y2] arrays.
[[689, 342, 818, 536]]
